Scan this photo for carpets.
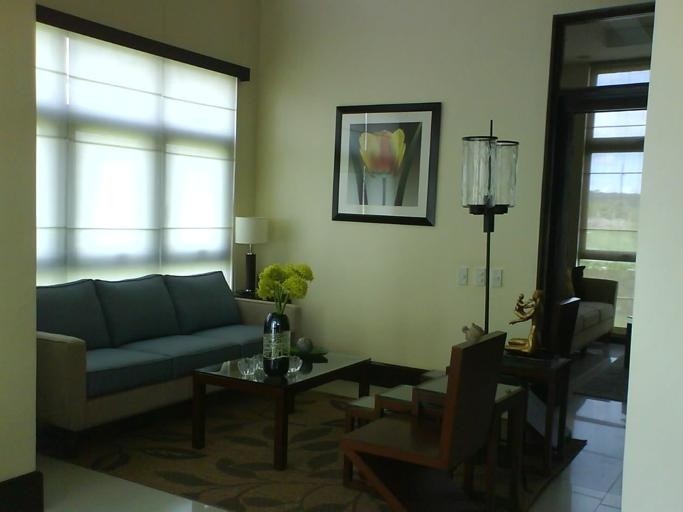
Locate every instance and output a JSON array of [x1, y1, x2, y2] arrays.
[[34, 379, 589, 511], [554, 342, 628, 405]]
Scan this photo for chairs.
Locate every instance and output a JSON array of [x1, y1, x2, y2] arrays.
[[339, 331, 504, 509], [444, 298, 580, 476]]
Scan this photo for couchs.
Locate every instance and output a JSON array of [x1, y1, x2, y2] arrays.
[[36, 270, 302, 468], [547, 255, 618, 360]]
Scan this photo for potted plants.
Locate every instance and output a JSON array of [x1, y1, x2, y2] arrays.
[[253, 261, 313, 376]]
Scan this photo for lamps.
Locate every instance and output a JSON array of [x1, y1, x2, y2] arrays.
[[235, 216, 268, 295], [459, 117, 519, 341]]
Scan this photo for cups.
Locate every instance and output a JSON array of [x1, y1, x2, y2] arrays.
[[236, 352, 305, 377]]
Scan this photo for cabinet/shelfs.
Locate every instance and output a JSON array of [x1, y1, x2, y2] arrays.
[[344, 377, 523, 508]]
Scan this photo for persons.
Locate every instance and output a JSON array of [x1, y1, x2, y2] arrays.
[[508, 289, 545, 354], [515, 293, 535, 317]]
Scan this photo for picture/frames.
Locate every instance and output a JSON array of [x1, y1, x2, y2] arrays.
[[332, 100, 441, 227]]
